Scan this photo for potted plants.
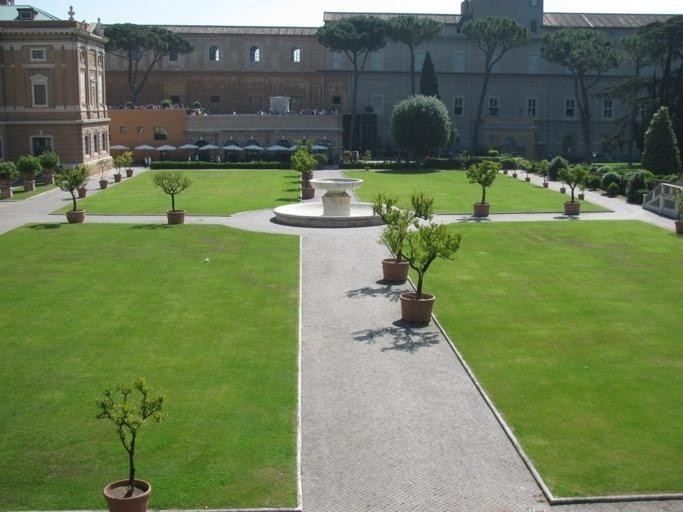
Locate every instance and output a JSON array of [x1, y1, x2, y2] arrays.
[[289, 145, 319, 198], [369, 189, 465, 324], [0, 149, 60, 199], [509, 156, 595, 216], [466, 159, 504, 217], [150, 171, 194, 225], [94, 377, 170, 510], [52, 150, 134, 224]]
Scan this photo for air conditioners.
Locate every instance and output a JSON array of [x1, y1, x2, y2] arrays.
[[671, 188, 682, 234]]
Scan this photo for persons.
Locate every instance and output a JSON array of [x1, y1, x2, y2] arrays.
[[108, 101, 162, 109], [300, 106, 339, 115]]
